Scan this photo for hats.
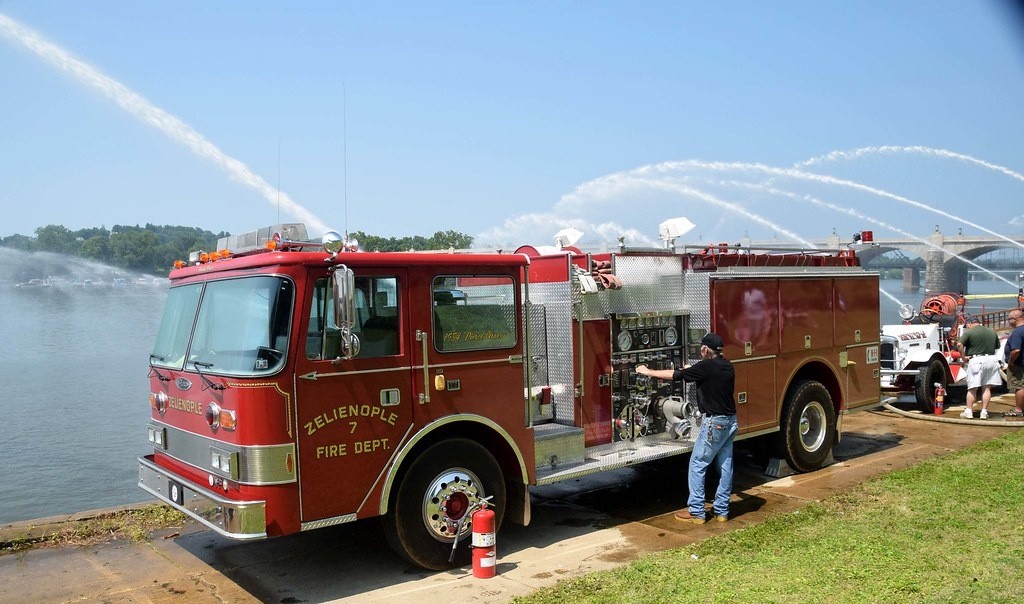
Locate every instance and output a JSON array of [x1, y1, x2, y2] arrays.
[[962, 316, 978, 328], [702, 333, 723, 351]]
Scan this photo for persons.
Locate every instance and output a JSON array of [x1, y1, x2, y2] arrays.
[[635, 333, 739, 524], [1002, 309, 1024, 418], [956, 317, 1003, 420]]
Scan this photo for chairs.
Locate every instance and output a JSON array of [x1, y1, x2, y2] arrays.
[[350, 316, 400, 358]]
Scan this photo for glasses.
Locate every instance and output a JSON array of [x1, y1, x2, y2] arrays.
[[1008, 317, 1018, 322]]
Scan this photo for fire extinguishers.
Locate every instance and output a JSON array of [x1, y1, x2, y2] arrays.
[[448, 495, 497, 579], [934, 382, 948, 415]]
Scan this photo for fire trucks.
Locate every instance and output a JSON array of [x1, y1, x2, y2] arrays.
[[138, 217, 881, 571]]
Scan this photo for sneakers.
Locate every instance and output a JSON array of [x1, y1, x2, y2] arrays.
[[710, 506, 728, 522], [960, 409, 973, 418], [674, 511, 705, 524], [979, 412, 989, 419]]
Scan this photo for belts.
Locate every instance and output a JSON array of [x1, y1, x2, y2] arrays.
[[971, 353, 994, 358]]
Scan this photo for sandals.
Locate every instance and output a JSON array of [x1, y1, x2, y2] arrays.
[[1000, 408, 1023, 418]]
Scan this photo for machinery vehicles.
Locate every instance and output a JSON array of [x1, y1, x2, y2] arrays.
[[880, 291, 1013, 412]]
